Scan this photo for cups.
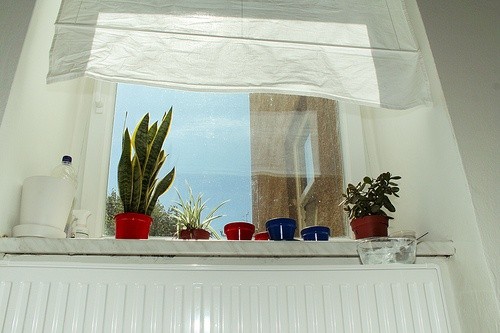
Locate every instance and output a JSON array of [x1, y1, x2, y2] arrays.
[[254, 232, 268, 240], [20, 173, 76, 231], [301, 225, 330, 241], [265, 218, 296, 241], [389, 230, 416, 237], [224, 222, 255, 240]]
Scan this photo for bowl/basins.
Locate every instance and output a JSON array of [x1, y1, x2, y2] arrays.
[[357, 236, 416, 264]]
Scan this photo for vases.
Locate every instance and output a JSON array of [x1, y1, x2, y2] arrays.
[[12, 176, 76, 238], [223, 217, 331, 241]]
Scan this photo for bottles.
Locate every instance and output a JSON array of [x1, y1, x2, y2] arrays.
[[52, 155, 78, 184]]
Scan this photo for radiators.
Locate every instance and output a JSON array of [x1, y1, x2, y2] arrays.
[[0, 260, 451, 333]]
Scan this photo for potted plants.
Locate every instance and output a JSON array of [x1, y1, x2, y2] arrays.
[[113, 104, 175, 239], [337, 172, 401, 240], [168, 180, 231, 239]]
